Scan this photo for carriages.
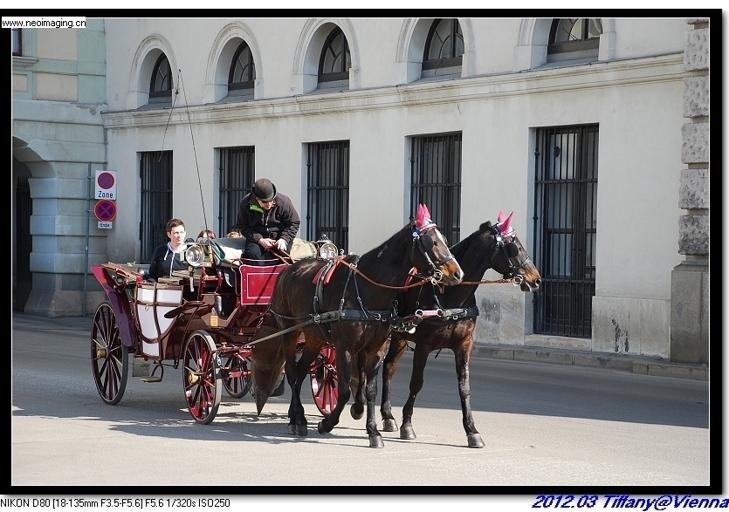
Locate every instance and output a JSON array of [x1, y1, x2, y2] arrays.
[[88, 202, 544, 448]]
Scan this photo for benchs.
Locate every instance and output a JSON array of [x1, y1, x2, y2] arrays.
[[203, 237, 320, 294]]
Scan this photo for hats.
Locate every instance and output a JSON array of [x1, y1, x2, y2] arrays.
[[250, 178, 278, 201]]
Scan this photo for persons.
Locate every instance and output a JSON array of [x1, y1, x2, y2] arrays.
[[226, 231, 242, 240], [236, 174, 301, 266], [198, 229, 217, 240], [146, 218, 231, 316]]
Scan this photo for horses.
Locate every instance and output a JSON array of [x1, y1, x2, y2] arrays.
[[350, 209, 543, 449], [252, 202, 465, 448]]
